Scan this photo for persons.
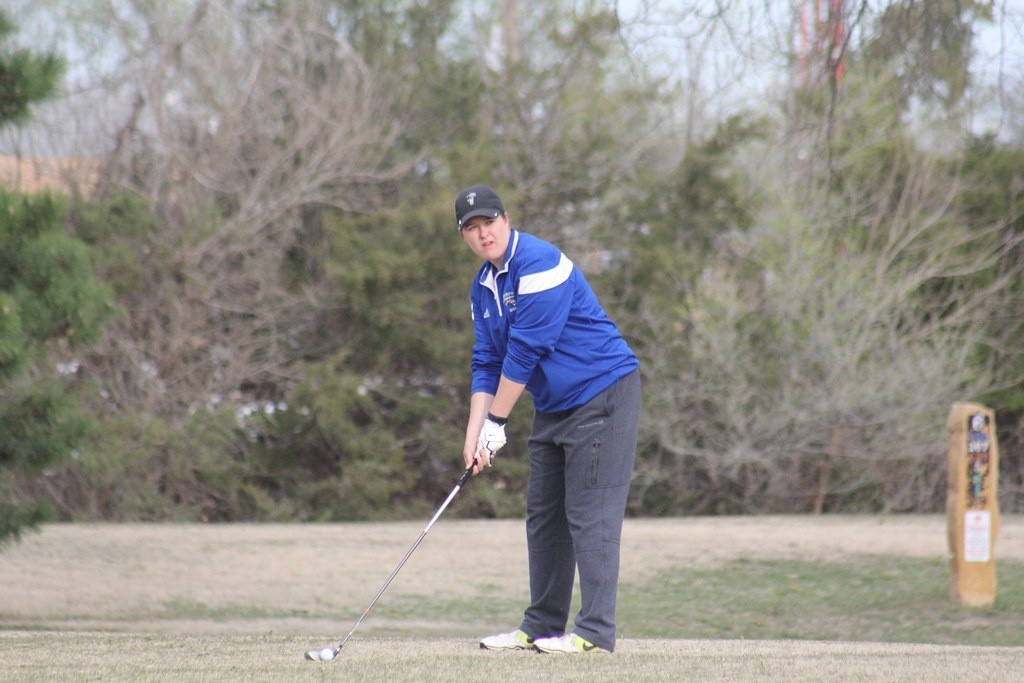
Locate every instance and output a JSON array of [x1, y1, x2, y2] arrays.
[[454, 187, 641, 655]]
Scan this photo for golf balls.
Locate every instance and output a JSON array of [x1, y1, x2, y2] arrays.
[[320, 649, 334, 661]]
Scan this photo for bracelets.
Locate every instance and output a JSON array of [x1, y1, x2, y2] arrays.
[[487, 412, 509, 425]]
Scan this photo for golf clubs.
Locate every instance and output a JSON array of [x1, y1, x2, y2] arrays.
[[304, 459, 478, 662]]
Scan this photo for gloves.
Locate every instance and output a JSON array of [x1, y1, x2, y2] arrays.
[[474, 413, 508, 467]]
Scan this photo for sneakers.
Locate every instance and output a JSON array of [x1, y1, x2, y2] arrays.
[[479, 629, 538, 650], [535, 632, 612, 654]]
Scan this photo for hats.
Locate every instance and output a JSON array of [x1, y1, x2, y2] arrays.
[[455, 186, 504, 231]]
